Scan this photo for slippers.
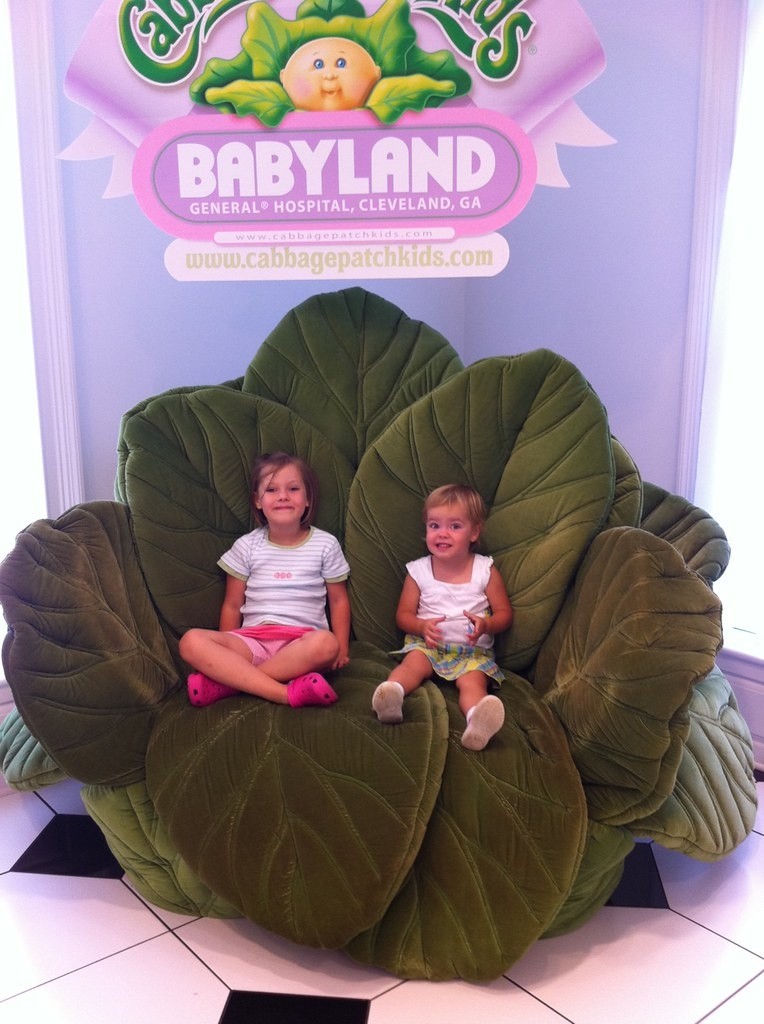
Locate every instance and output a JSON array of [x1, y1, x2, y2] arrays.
[[186, 671, 240, 708], [286, 672, 337, 708]]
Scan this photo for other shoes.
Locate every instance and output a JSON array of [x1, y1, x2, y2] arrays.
[[371, 681, 404, 723], [460, 695, 505, 752]]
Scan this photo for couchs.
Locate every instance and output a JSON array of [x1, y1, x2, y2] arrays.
[[0, 284, 761, 986]]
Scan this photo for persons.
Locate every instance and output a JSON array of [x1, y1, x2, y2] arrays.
[[372, 482, 512, 751], [179, 453, 350, 708]]
